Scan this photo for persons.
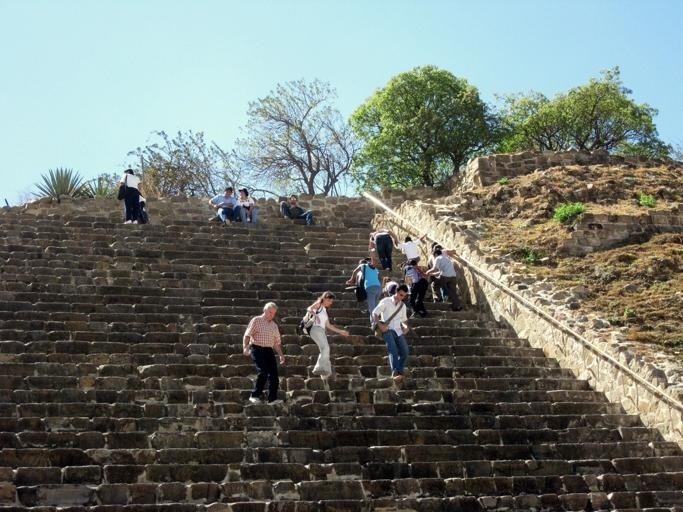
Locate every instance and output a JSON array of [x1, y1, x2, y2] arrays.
[[209, 187, 242, 226], [120, 169, 139, 224], [243, 302, 285, 405], [139, 195, 149, 224], [280, 194, 312, 226], [237, 188, 256, 224], [346, 229, 462, 328], [372, 285, 410, 379], [302, 291, 349, 378]]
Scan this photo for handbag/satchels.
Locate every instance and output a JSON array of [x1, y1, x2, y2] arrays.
[[344, 284, 368, 303], [372, 321, 386, 342], [117, 173, 129, 200]]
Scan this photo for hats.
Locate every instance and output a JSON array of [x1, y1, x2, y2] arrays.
[[239, 188, 251, 196]]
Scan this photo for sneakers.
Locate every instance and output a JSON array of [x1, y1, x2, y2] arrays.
[[248, 394, 263, 406], [389, 369, 407, 381], [411, 311, 432, 320], [131, 220, 138, 225], [124, 219, 133, 224], [267, 398, 285, 407], [224, 219, 234, 228], [430, 296, 464, 311], [311, 368, 329, 376], [321, 372, 336, 381]]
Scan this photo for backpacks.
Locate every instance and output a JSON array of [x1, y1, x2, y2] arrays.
[[294, 301, 323, 338]]
